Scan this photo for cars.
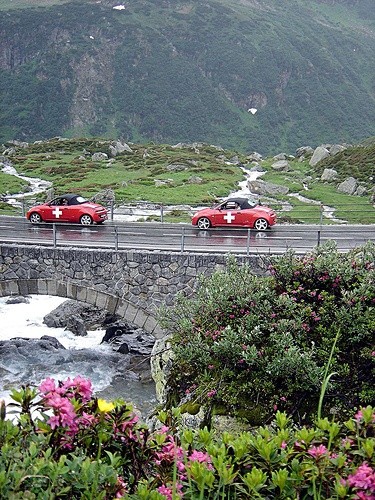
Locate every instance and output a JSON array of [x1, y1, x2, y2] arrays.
[[27, 194, 108, 227], [191, 198, 276, 231]]
